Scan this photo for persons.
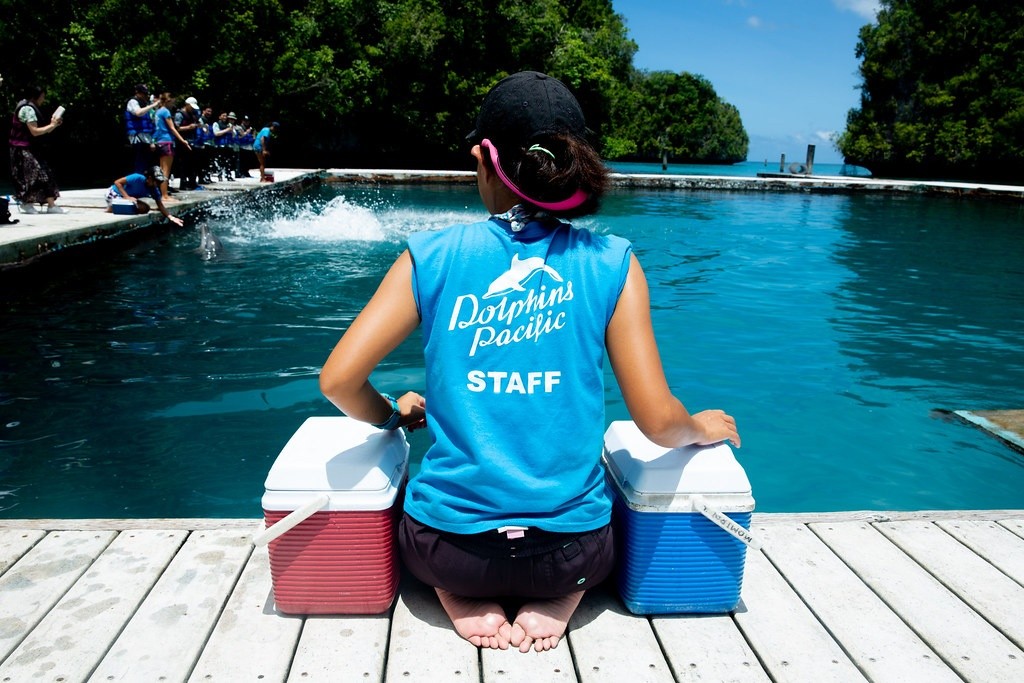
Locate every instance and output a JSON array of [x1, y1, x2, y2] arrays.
[[103, 82, 282, 227], [9, 85, 70, 215], [317, 69, 742, 653]]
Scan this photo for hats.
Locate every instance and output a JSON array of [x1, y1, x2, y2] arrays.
[[147, 165, 168, 181], [242, 115, 250, 121], [185, 97, 200, 110], [272, 122, 280, 127], [464, 70, 596, 145], [227, 112, 237, 120], [135, 84, 151, 93]]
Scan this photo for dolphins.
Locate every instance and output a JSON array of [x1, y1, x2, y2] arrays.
[[193, 222, 224, 260]]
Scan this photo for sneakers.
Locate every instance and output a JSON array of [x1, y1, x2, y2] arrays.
[[20, 203, 39, 214], [48, 206, 69, 214]]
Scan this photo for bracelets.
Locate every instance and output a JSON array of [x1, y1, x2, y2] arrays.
[[170, 217, 175, 221], [370, 392, 401, 431]]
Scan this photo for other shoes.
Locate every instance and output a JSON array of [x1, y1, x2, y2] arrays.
[[198, 173, 216, 185], [180, 181, 204, 191]]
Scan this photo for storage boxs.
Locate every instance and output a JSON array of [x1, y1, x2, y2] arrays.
[[600, 418, 756, 617], [111, 198, 139, 215], [261, 416, 411, 615]]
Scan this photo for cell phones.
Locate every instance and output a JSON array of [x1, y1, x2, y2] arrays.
[[52, 106, 65, 119]]
